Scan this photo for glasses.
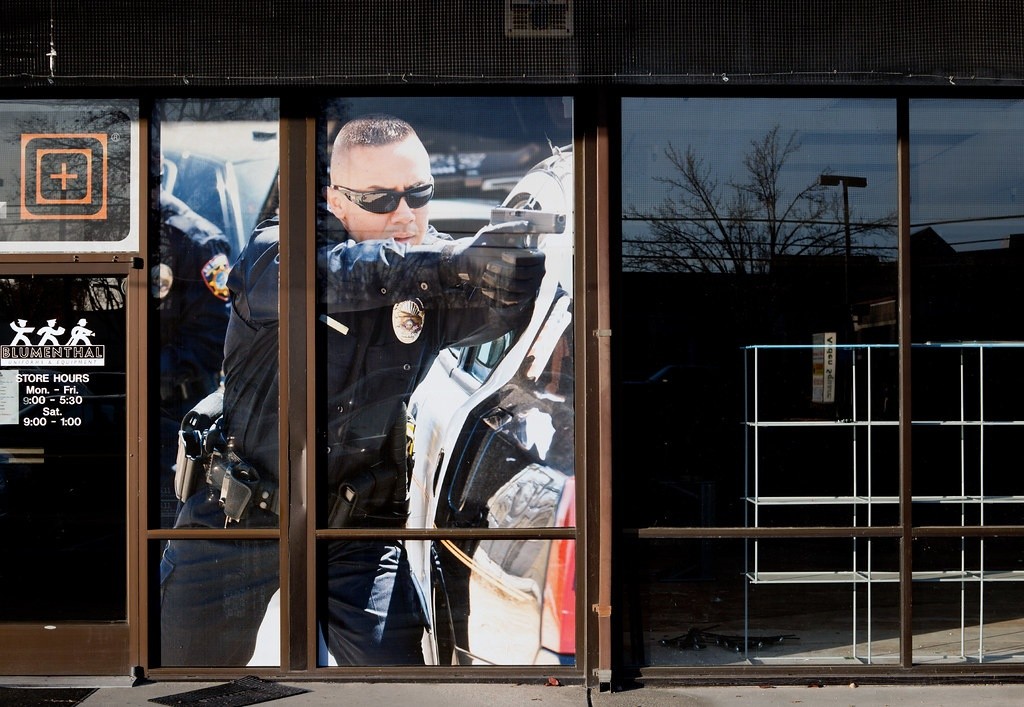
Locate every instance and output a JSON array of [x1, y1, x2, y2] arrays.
[[334, 177, 435, 214]]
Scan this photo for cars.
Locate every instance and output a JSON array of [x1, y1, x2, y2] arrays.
[[159, 120, 282, 270], [397, 152, 578, 668]]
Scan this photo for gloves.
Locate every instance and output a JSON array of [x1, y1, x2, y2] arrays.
[[439, 218, 533, 311], [470, 250, 546, 333]]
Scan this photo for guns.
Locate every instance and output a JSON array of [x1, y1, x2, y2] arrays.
[[489, 208, 566, 234]]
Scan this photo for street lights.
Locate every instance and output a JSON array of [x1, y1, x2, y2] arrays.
[[815, 174, 869, 271]]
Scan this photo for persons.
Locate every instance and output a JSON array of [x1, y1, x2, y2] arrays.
[[160, 115, 545, 665], [161, 100, 277, 545]]
